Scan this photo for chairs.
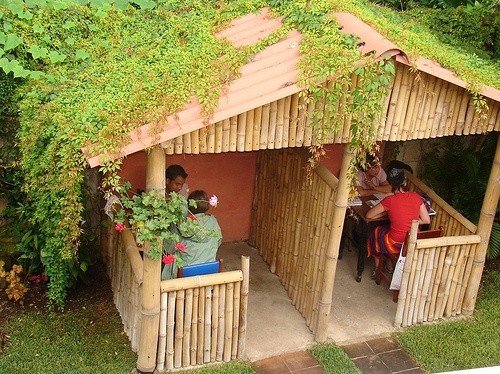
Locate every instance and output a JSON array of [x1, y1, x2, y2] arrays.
[[178, 259, 223, 278], [375, 226, 444, 303]]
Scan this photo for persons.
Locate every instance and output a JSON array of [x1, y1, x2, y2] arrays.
[[163, 165, 188, 215], [161, 190, 222, 280], [351, 151, 392, 196], [367, 168, 430, 281]]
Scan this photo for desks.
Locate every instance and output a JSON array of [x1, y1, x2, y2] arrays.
[[338, 194, 437, 283]]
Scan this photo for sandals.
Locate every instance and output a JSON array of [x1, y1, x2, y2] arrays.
[[383, 262, 393, 273], [371, 267, 378, 279]]
